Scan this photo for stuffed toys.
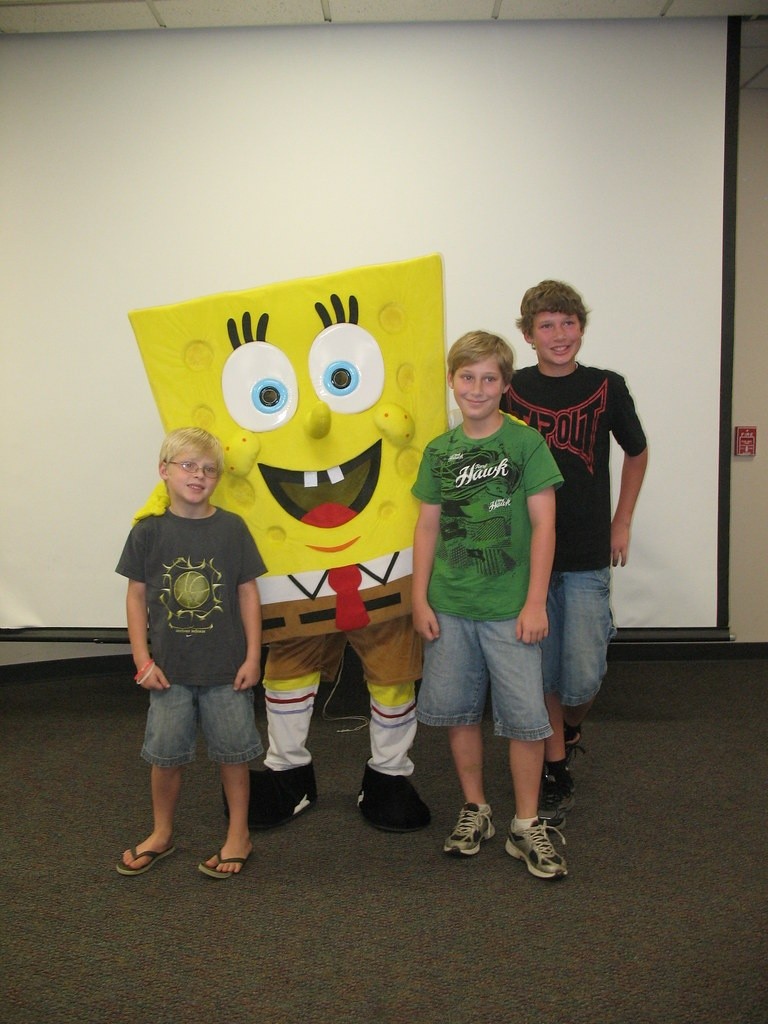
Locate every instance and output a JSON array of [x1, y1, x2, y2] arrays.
[[126, 253, 529, 835]]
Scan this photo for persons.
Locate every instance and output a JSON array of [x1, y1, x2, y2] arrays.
[[409, 332, 569, 882], [115, 430, 268, 880], [498, 280, 647, 833]]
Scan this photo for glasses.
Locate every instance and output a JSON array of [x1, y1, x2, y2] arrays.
[[165, 460, 220, 479]]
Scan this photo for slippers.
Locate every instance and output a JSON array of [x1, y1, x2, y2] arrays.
[[116, 845, 175, 875], [198, 848, 248, 878]]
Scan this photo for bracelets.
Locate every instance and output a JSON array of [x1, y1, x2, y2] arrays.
[[133, 660, 156, 684]]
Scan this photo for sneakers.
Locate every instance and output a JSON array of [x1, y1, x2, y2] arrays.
[[536, 772, 575, 832], [504, 819, 568, 878], [444, 803, 494, 855], [565, 732, 586, 764]]
